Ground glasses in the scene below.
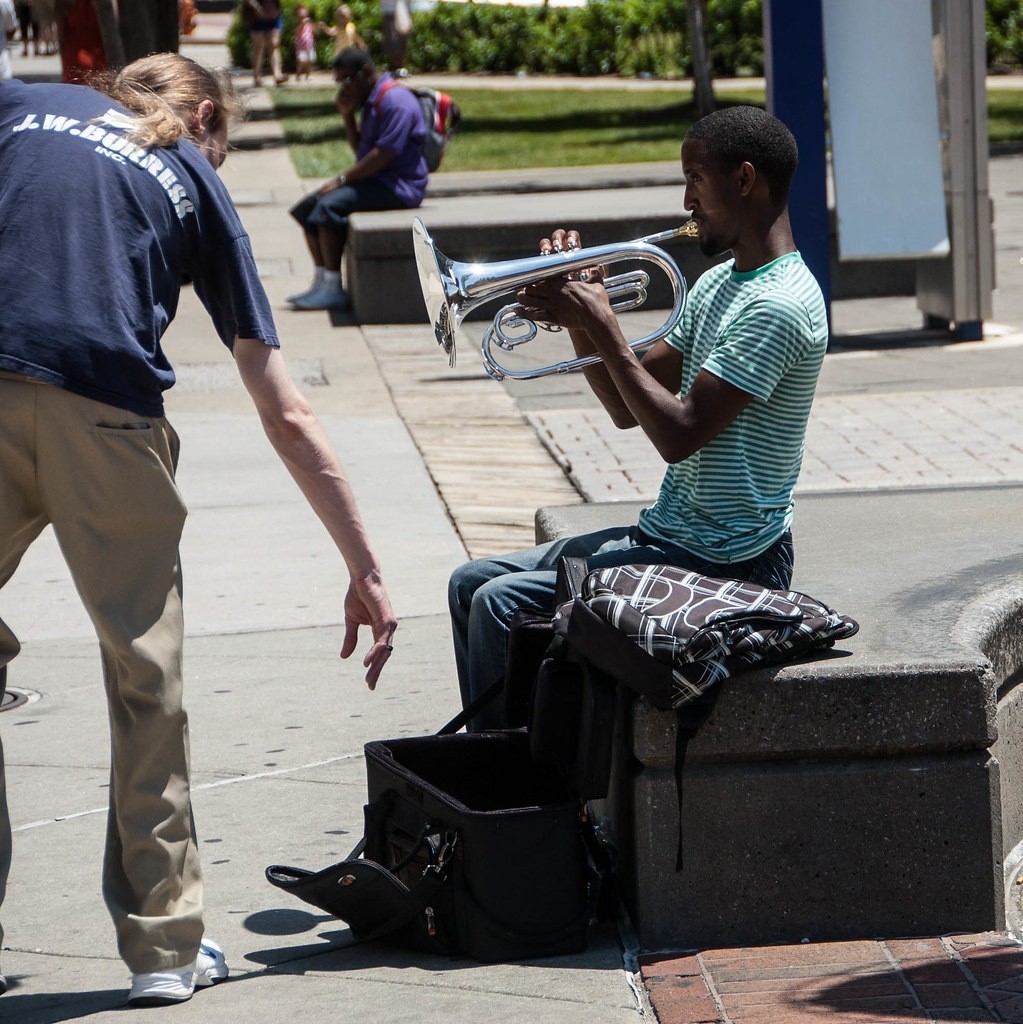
[337,64,364,87]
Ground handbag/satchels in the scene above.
[569,558,859,715]
[266,598,620,961]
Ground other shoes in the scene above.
[288,281,347,310]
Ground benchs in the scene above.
[342,158,732,323]
[537,481,1023,950]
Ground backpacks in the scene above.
[373,80,458,172]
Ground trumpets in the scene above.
[406,216,701,382]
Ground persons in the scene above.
[1,53,397,1000]
[1,0,59,57]
[289,49,431,310]
[447,106,827,731]
[247,1,412,87]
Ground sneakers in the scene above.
[127,938,230,1002]
